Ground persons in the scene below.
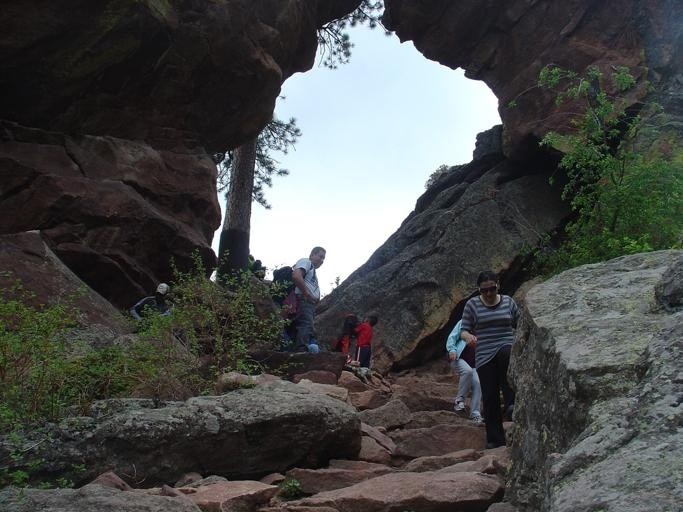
[284,247,326,351]
[460,271,520,449]
[446,319,483,423]
[129,283,176,323]
[352,315,378,367]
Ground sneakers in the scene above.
[470,410,486,424]
[454,395,465,412]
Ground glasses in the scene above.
[480,287,497,292]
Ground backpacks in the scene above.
[270,258,314,304]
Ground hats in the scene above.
[156,283,170,295]
